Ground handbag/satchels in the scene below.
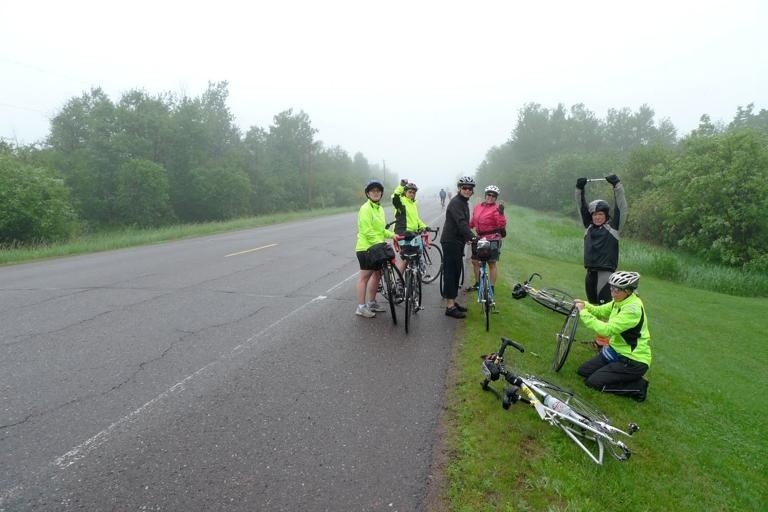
[365,244,395,268]
[472,240,500,262]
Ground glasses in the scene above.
[610,285,622,292]
[487,193,496,197]
[462,186,472,190]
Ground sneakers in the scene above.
[445,302,468,319]
[356,305,376,318]
[366,302,386,312]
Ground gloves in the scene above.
[605,174,620,186]
[496,204,504,214]
[577,177,588,187]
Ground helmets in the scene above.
[404,182,418,190]
[608,270,640,290]
[365,181,384,193]
[589,199,609,215]
[484,185,501,195]
[457,176,475,188]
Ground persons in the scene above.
[440,177,474,319]
[439,188,446,206]
[463,186,506,293]
[391,179,430,283]
[574,272,652,402]
[448,190,452,199]
[351,181,405,319]
[575,173,627,305]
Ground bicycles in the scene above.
[378,221,465,333]
[512,272,581,317]
[478,335,639,467]
[465,233,502,332]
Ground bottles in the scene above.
[543,393,570,419]
